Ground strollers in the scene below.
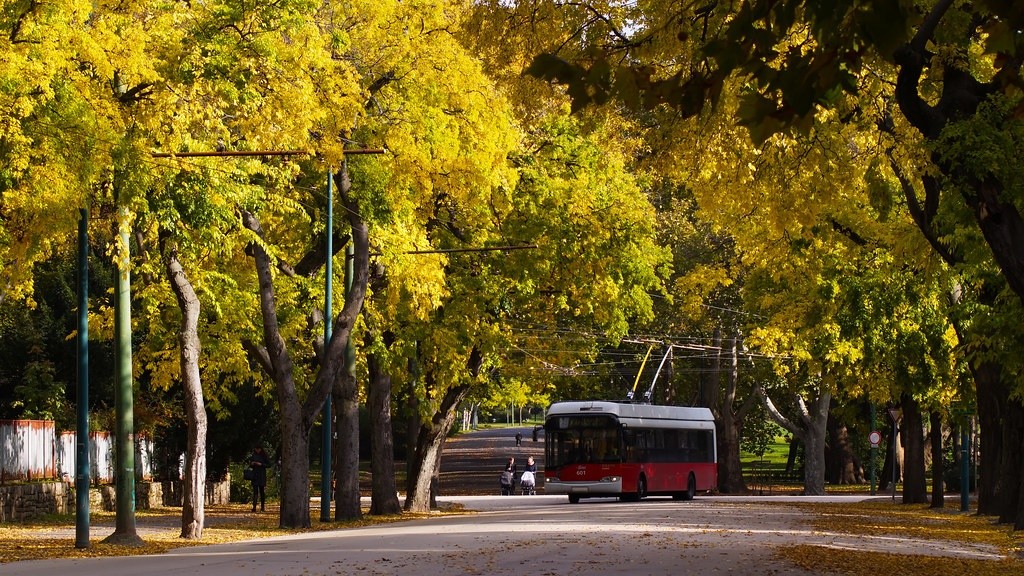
[520,471,536,495]
[500,471,512,495]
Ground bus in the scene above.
[543,402,718,503]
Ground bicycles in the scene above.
[516,439,521,450]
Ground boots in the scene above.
[261,502,264,511]
[251,503,256,511]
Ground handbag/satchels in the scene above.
[243,466,258,480]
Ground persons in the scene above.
[249,459,267,512]
[523,456,537,495]
[505,457,517,495]
[515,432,522,447]
